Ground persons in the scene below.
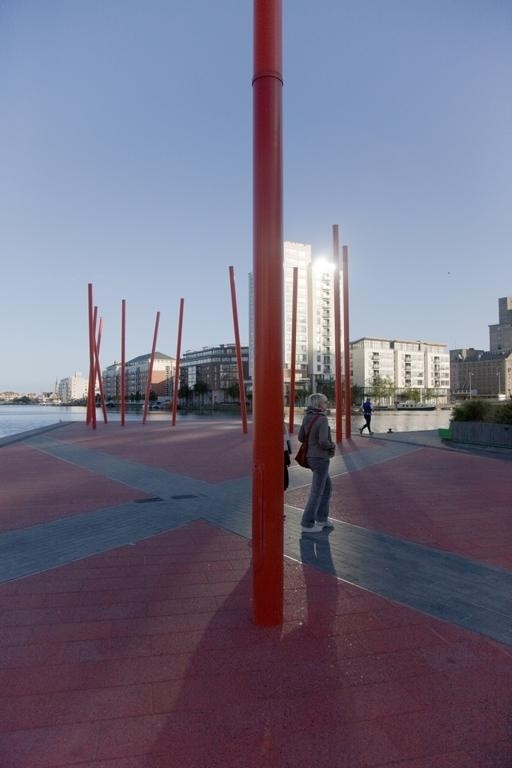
[283,410,292,517]
[298,393,336,532]
[358,397,374,435]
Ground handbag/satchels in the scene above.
[294,441,311,468]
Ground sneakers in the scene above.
[369,432,374,435]
[359,429,362,436]
[302,526,323,532]
[315,521,332,527]
